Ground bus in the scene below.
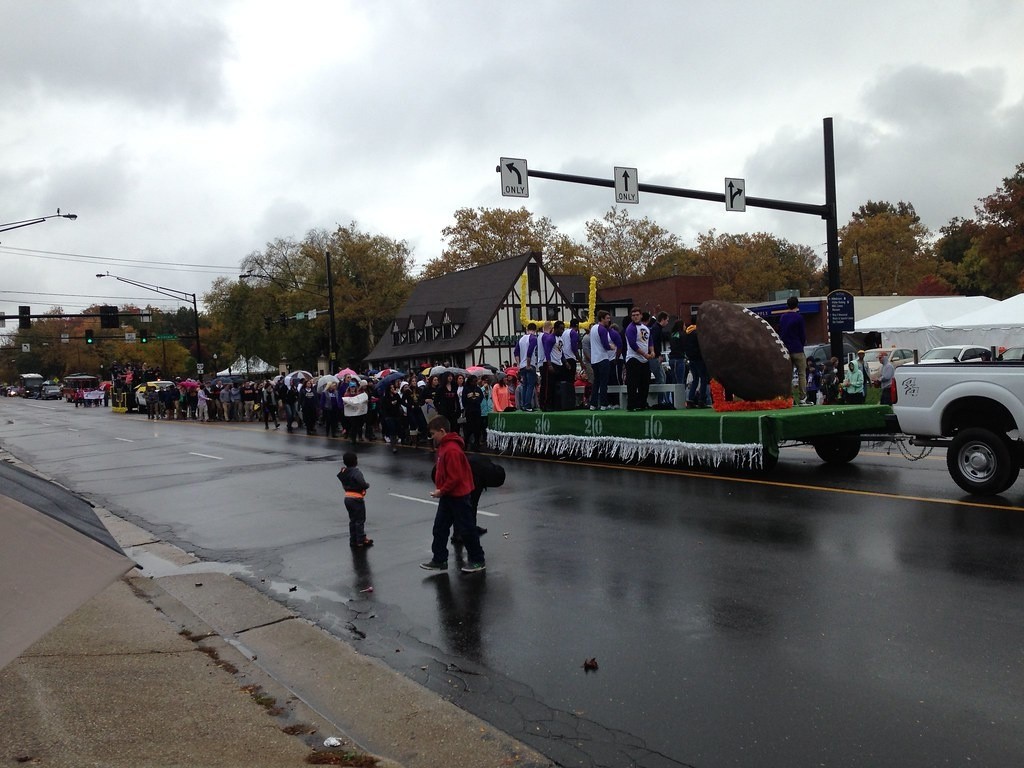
[63,373,100,404]
[19,374,44,399]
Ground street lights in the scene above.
[96,270,205,384]
[238,270,339,376]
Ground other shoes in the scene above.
[698,402,712,408]
[350,538,374,548]
[477,526,487,535]
[451,534,463,543]
[590,405,596,411]
[686,400,697,408]
[265,420,432,452]
[600,405,607,410]
[522,406,538,411]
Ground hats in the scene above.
[876,352,887,358]
[857,350,865,355]
[359,379,369,386]
[497,372,506,382]
[417,381,426,388]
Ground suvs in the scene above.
[802,343,858,371]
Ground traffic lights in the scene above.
[85,329,94,345]
[139,329,148,343]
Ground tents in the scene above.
[215,353,279,380]
[854,293,1024,360]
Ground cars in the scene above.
[134,380,175,414]
[903,345,992,365]
[1000,346,1024,362]
[41,386,63,400]
[844,347,923,384]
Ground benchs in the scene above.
[576,384,686,410]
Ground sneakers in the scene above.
[420,557,449,570]
[461,559,485,572]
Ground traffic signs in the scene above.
[499,157,529,197]
[613,166,640,204]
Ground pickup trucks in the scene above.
[891,359,1024,498]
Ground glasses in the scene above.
[346,376,351,379]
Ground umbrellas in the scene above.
[338,368,357,377]
[285,370,312,379]
[373,372,409,391]
[442,367,467,375]
[317,375,339,393]
[374,369,399,378]
[465,366,495,376]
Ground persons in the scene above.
[74,382,111,408]
[513,323,538,412]
[581,307,677,411]
[336,452,373,547]
[420,415,488,572]
[670,316,713,409]
[537,318,587,412]
[112,358,541,453]
[778,296,815,407]
[804,349,894,407]
